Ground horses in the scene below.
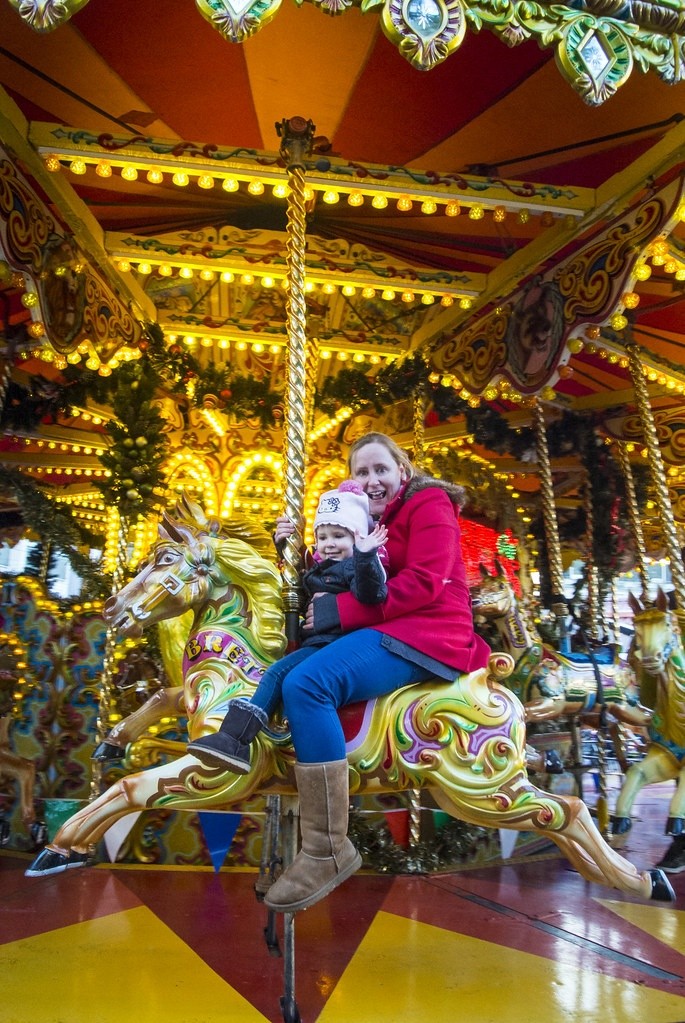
[468,559,656,775]
[613,584,685,835]
[25,491,677,905]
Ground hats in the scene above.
[313,479,370,539]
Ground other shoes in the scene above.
[654,833,685,873]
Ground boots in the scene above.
[263,759,362,913]
[187,698,268,774]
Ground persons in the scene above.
[185,478,393,776]
[254,432,492,913]
